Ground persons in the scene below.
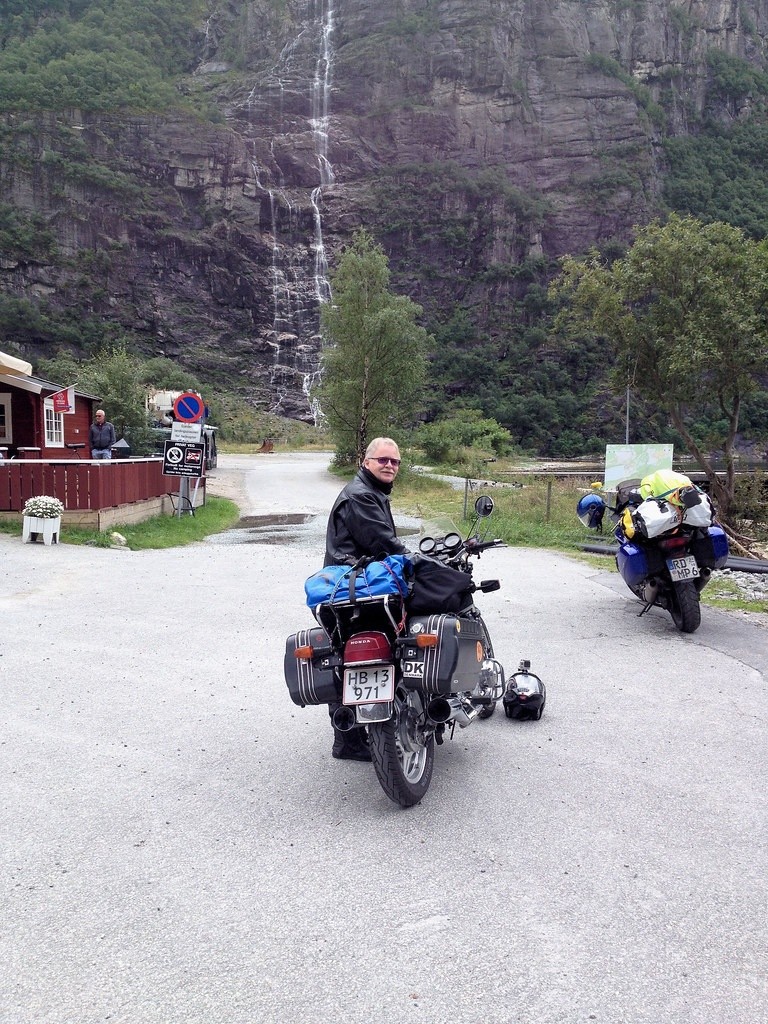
[323,438,411,763]
[88,410,116,459]
[587,504,600,527]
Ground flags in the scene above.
[53,385,75,414]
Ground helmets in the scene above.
[503,659,546,721]
[577,494,606,533]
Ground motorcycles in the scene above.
[576,478,729,633]
[284,495,508,807]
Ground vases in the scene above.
[22,516,61,546]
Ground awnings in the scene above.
[0,351,32,376]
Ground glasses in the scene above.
[95,414,101,417]
[368,457,401,467]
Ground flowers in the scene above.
[21,494,65,519]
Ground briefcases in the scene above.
[402,614,485,693]
[284,627,343,708]
